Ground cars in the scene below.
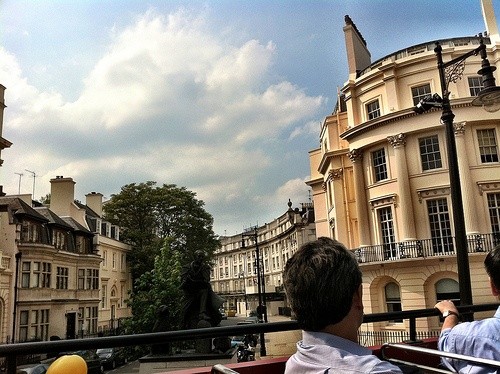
[15,364,51,374]
[96,347,125,369]
[230,335,246,346]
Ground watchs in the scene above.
[441,310,460,319]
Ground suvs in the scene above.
[56,350,104,374]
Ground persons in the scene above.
[434,243,500,374]
[283,235,404,374]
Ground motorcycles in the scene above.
[237,343,257,362]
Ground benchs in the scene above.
[381,343,500,374]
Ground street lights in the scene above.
[433,34,500,325]
[242,234,267,321]
